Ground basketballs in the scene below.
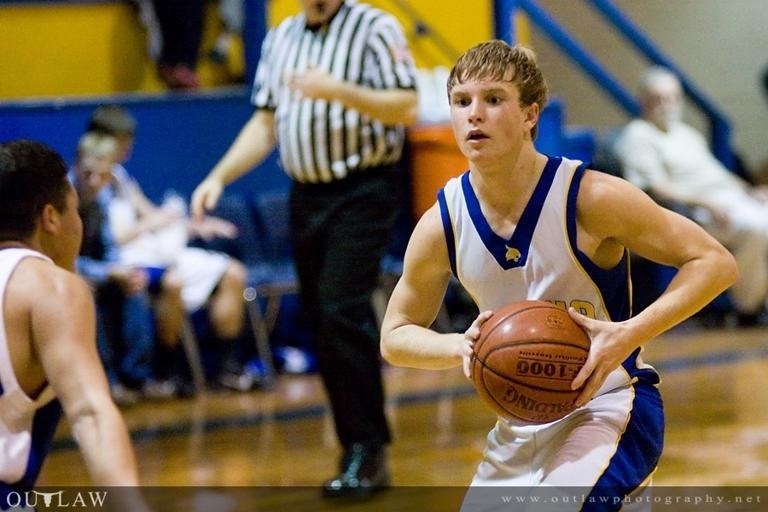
[468,300,591,425]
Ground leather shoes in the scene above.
[324,440,391,499]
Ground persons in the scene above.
[191,0,420,505]
[66,108,262,406]
[132,0,211,92]
[610,66,768,329]
[0,138,149,512]
[377,40,740,512]
